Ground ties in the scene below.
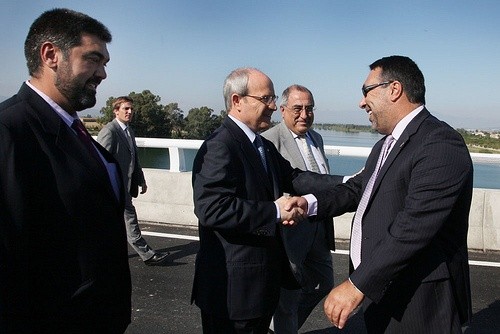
[349,135,393,270]
[125,127,135,170]
[299,134,321,174]
[255,135,268,176]
[71,118,107,172]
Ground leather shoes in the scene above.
[144,252,169,266]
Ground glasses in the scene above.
[283,104,316,114]
[240,95,278,106]
[362,80,401,98]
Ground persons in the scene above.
[190,68,366,334]
[97,97,170,265]
[258,84,358,334]
[283,56,474,334]
[0,9,132,334]
